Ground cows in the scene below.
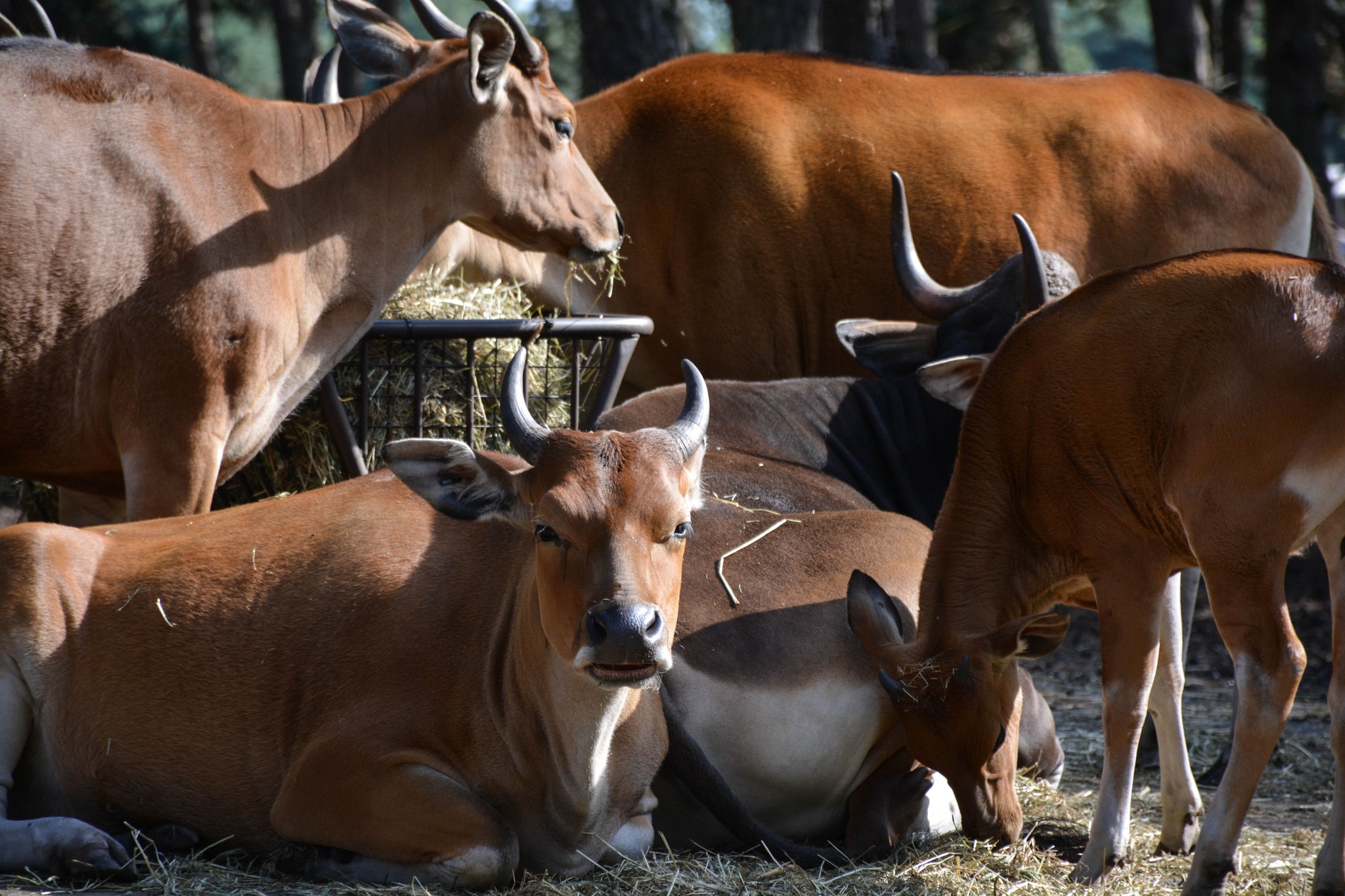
[0,0,621,529]
[271,0,1344,896]
[0,348,710,889]
